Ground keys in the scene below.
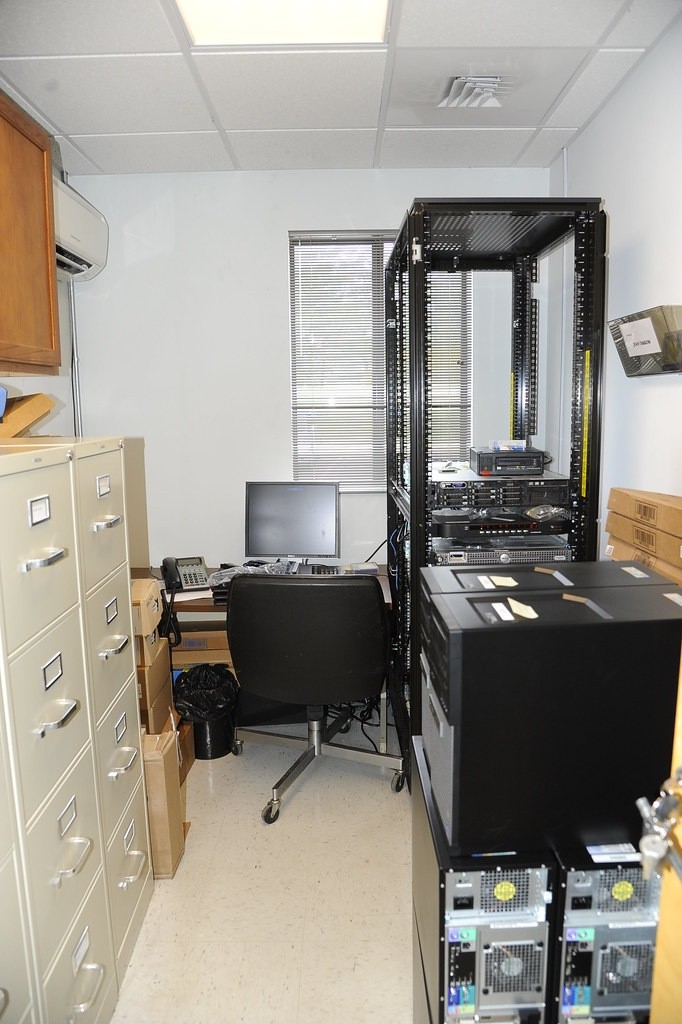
[639,834,668,880]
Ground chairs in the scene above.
[224,573,413,824]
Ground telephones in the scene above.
[160,556,211,592]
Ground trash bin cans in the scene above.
[173,663,237,762]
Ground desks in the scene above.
[129,564,229,611]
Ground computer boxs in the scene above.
[412,559,682,1024]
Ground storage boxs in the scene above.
[130,575,230,876]
[0,392,54,439]
[605,488,682,589]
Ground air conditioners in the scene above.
[53,172,109,284]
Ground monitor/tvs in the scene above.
[245,482,340,564]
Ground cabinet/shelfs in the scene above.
[0,436,151,1024]
[0,86,61,378]
